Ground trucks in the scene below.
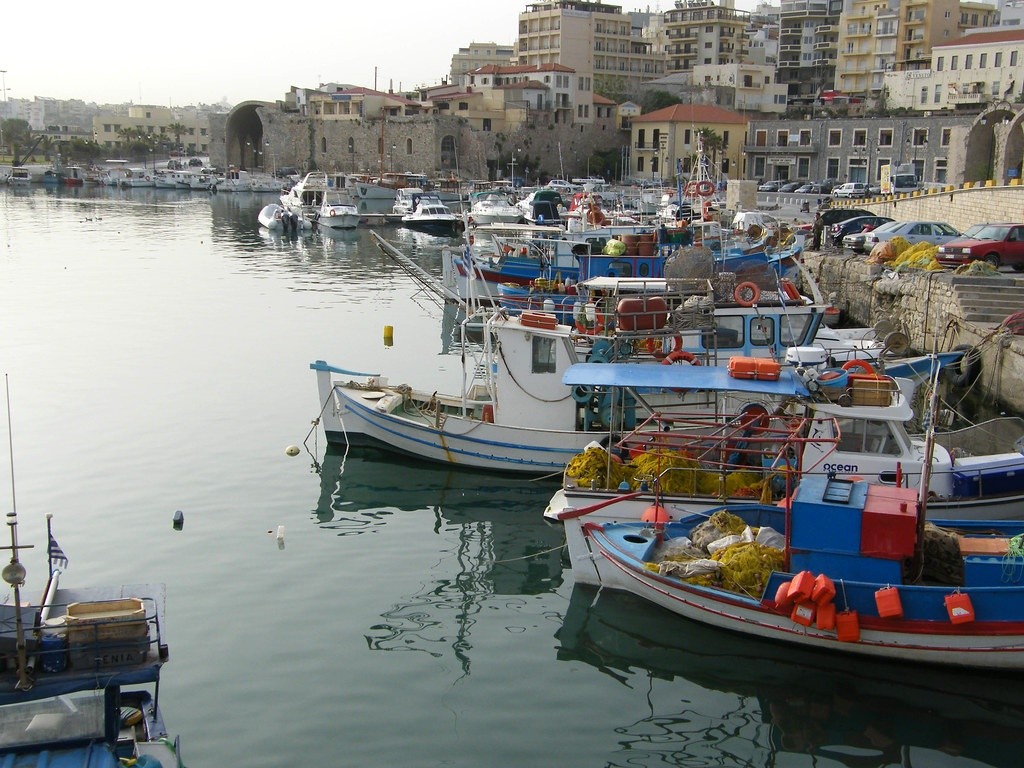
[880,163,920,200]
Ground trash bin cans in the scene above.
[336,176,346,188]
[802,202,809,211]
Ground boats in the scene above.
[0,371,186,768]
[6,166,33,187]
[44,125,1024,673]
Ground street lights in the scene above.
[348,142,355,174]
[387,141,397,173]
[905,127,931,181]
[861,137,881,198]
[511,145,522,188]
[737,140,747,180]
[258,133,271,173]
[852,143,867,183]
[246,138,256,175]
[655,141,664,187]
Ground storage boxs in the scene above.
[850,378,893,405]
[954,451,1024,498]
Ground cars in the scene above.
[820,207,877,226]
[840,220,942,255]
[935,222,1024,274]
[863,220,964,253]
[829,215,897,246]
